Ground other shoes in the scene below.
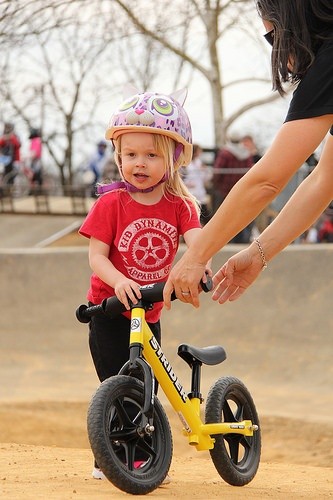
[92,460,171,485]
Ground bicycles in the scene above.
[75,269,262,496]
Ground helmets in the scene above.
[104,92,193,167]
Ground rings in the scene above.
[182,292,190,295]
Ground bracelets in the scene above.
[255,239,267,270]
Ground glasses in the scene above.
[263,27,275,47]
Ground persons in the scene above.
[78,93,212,481]
[0,124,45,195]
[162,0,333,309]
[89,141,111,198]
[184,136,333,241]
